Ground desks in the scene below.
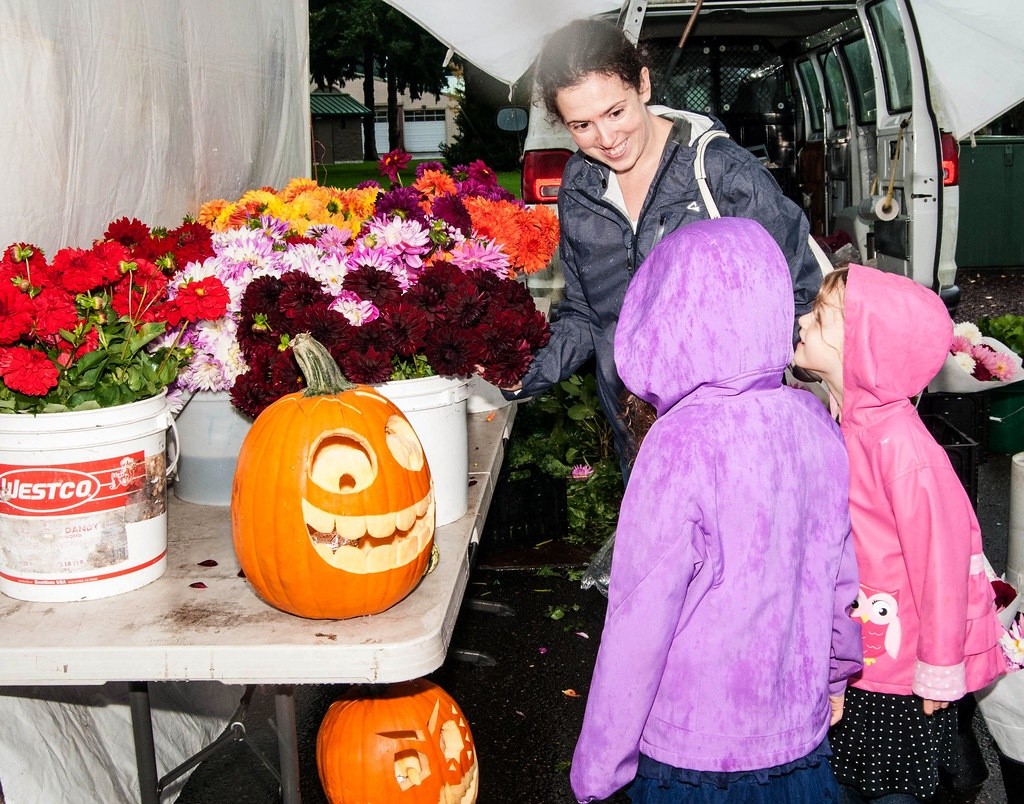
[0,404,516,804]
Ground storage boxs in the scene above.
[921,413,977,511]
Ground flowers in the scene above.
[0,149,560,412]
[931,322,1024,391]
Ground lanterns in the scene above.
[316,678,479,804]
[233,332,434,617]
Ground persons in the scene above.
[569,218,861,803]
[470,19,825,493]
[795,262,1005,804]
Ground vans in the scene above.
[495,0,960,319]
